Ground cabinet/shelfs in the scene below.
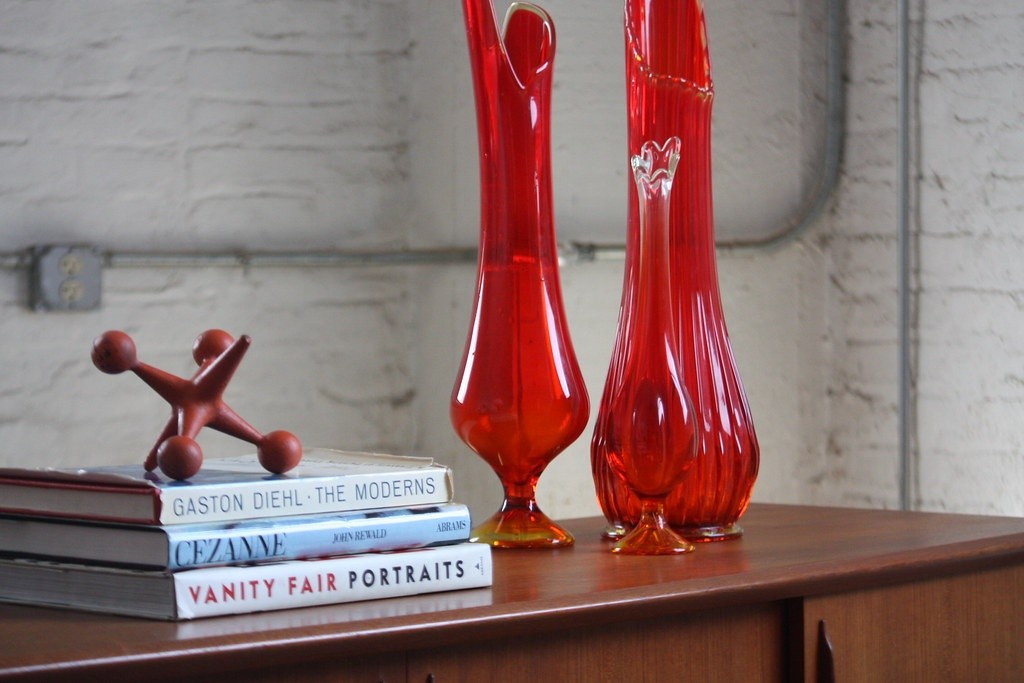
[0,504,1024,683]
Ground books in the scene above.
[0,447,493,622]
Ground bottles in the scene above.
[609,135,693,556]
[589,1,761,544]
[448,1,590,551]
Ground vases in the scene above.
[591,0,756,555]
[450,2,592,551]
